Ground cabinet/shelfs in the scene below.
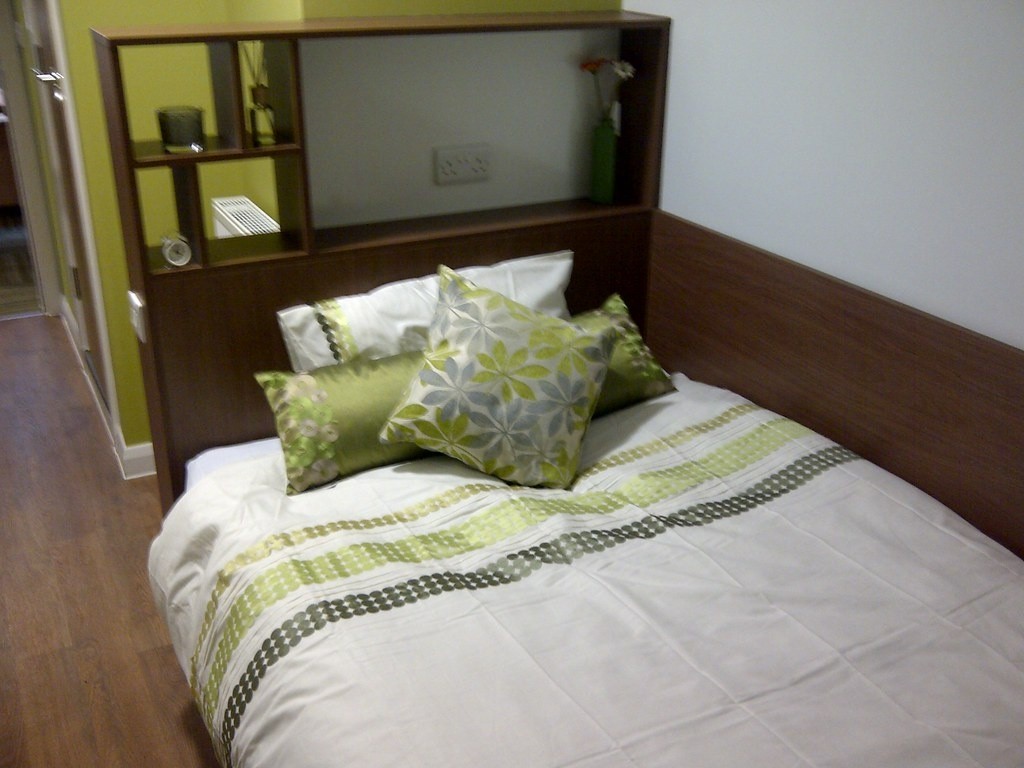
[91,9,672,517]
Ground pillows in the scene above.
[254,294,677,496]
[277,249,574,373]
[379,264,626,489]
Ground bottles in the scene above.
[247,84,283,150]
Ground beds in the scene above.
[146,372,1023,767]
